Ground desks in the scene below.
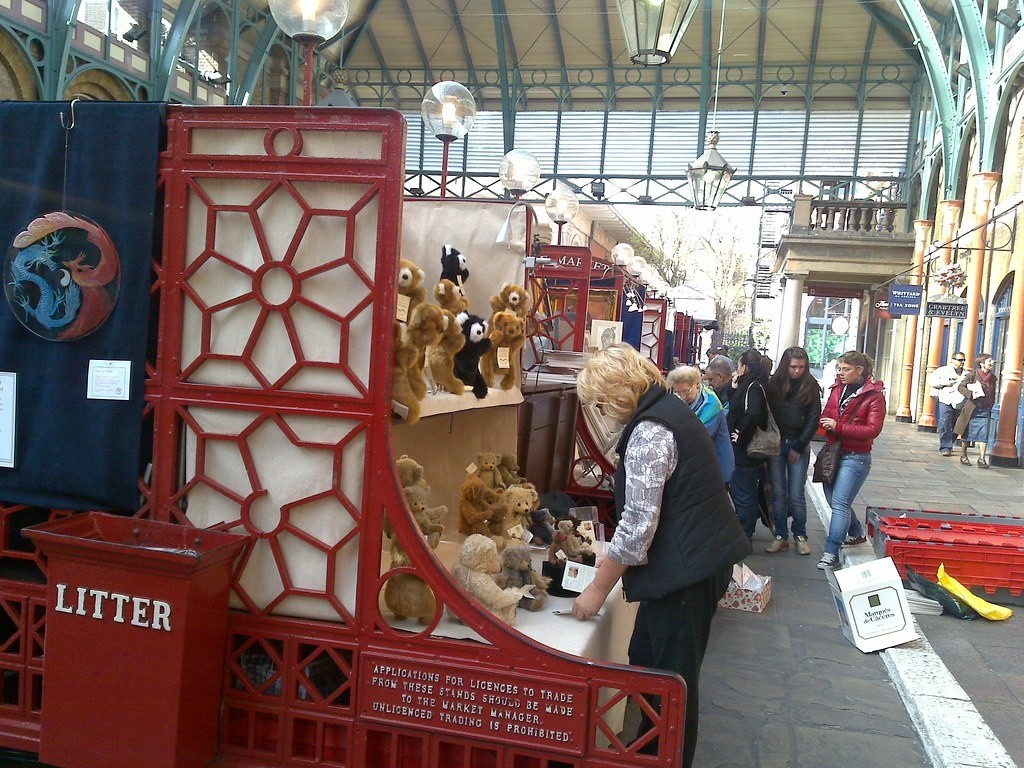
[378,540,641,749]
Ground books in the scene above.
[905,590,944,616]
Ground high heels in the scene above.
[977,458,990,468]
[960,456,971,466]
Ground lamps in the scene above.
[639,196,651,203]
[204,71,232,84]
[623,279,648,312]
[545,187,579,245]
[614,0,701,68]
[685,0,738,212]
[409,188,424,196]
[420,81,478,197]
[590,181,605,196]
[613,243,676,306]
[498,149,540,201]
[267,0,349,105]
[741,197,756,205]
[122,23,147,43]
[495,200,540,258]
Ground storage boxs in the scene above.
[864,505,1024,607]
[823,556,917,654]
[717,574,771,614]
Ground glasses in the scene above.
[953,357,966,362]
[837,365,861,373]
[596,401,604,409]
[676,384,695,396]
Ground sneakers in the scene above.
[795,536,811,555]
[766,534,789,552]
[841,534,868,548]
[817,552,839,570]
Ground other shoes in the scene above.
[941,451,950,455]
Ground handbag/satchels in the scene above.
[906,564,980,621]
[935,562,1013,621]
[812,442,841,485]
[744,381,782,459]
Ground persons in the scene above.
[816,351,886,570]
[701,345,777,537]
[572,342,752,768]
[764,346,823,555]
[665,365,735,484]
[932,352,970,456]
[953,354,998,467]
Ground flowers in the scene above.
[933,264,967,289]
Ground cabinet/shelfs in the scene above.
[0,105,698,768]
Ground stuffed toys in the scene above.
[385,451,596,625]
[391,244,531,425]
